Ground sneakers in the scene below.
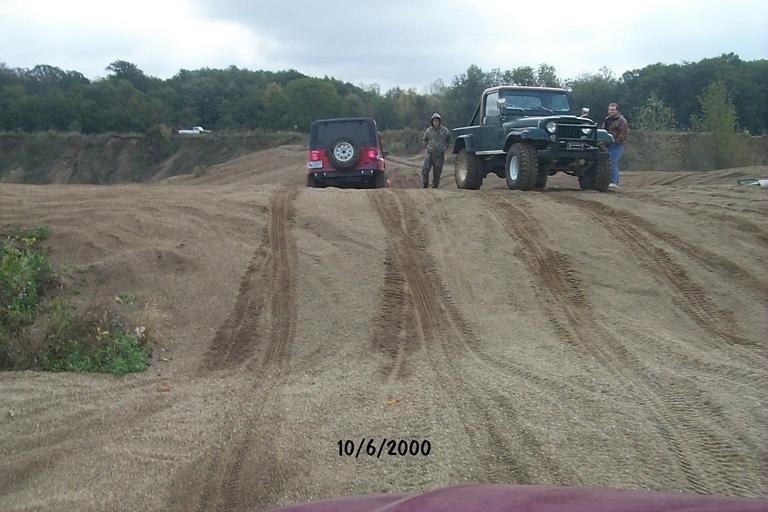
[608,182,618,188]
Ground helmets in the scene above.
[431,113,441,126]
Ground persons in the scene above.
[603,103,629,188]
[421,113,451,188]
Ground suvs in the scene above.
[305,116,388,189]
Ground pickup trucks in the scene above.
[179,126,211,136]
[449,85,616,196]
